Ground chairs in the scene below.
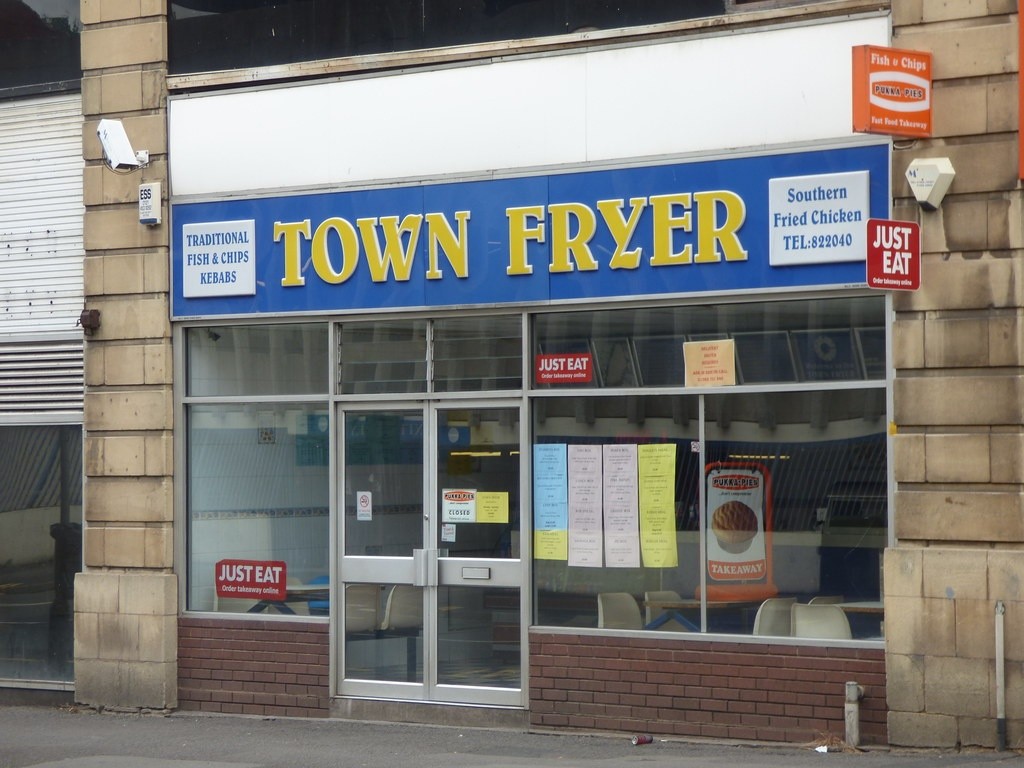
[751,593,855,642]
[595,590,692,634]
[307,574,421,681]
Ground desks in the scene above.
[825,602,886,640]
[249,584,327,615]
[645,599,763,635]
